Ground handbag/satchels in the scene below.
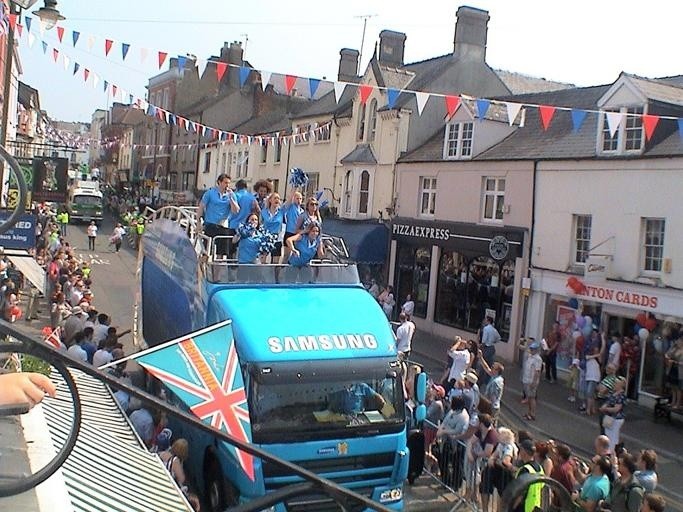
[602,414,615,430]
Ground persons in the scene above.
[196,173,324,283]
[365,275,415,364]
[379,329,682,512]
[329,383,385,414]
[544,321,561,383]
[0,162,201,512]
[477,316,502,361]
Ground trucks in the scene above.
[64,167,105,227]
[131,201,411,512]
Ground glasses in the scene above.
[310,202,318,206]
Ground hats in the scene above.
[157,429,172,446]
[518,440,536,452]
[62,289,98,319]
[431,384,446,398]
[463,372,478,384]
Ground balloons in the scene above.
[620,314,655,373]
[566,278,593,350]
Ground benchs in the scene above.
[654,377,683,421]
